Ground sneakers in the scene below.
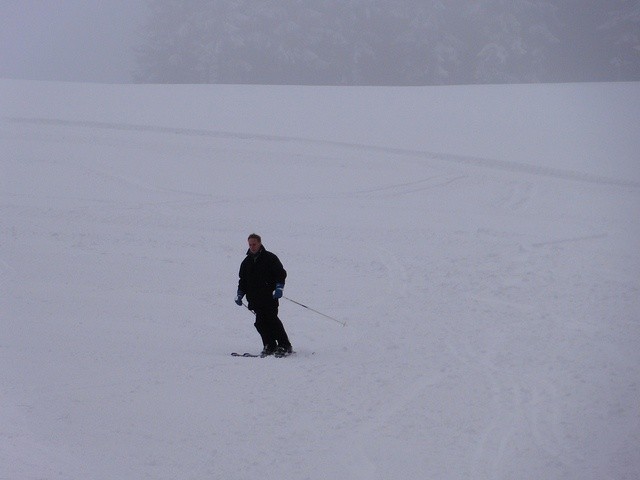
[261,343,277,355]
[275,345,291,356]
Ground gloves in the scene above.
[272,284,284,298]
[236,291,245,306]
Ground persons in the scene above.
[235,233,293,358]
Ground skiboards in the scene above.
[231,351,295,358]
